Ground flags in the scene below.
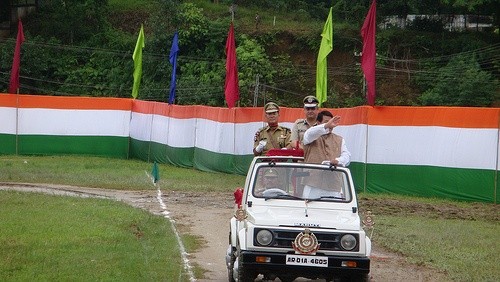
[132,24,145,99]
[225,24,240,109]
[361,0,376,106]
[9,18,25,94]
[316,7,333,108]
[169,31,179,104]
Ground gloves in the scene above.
[256,140,266,153]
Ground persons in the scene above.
[291,95,319,197]
[253,102,296,193]
[303,111,351,199]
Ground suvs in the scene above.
[226,149,373,282]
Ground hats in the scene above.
[264,102,280,113]
[303,95,319,107]
[263,168,280,177]
